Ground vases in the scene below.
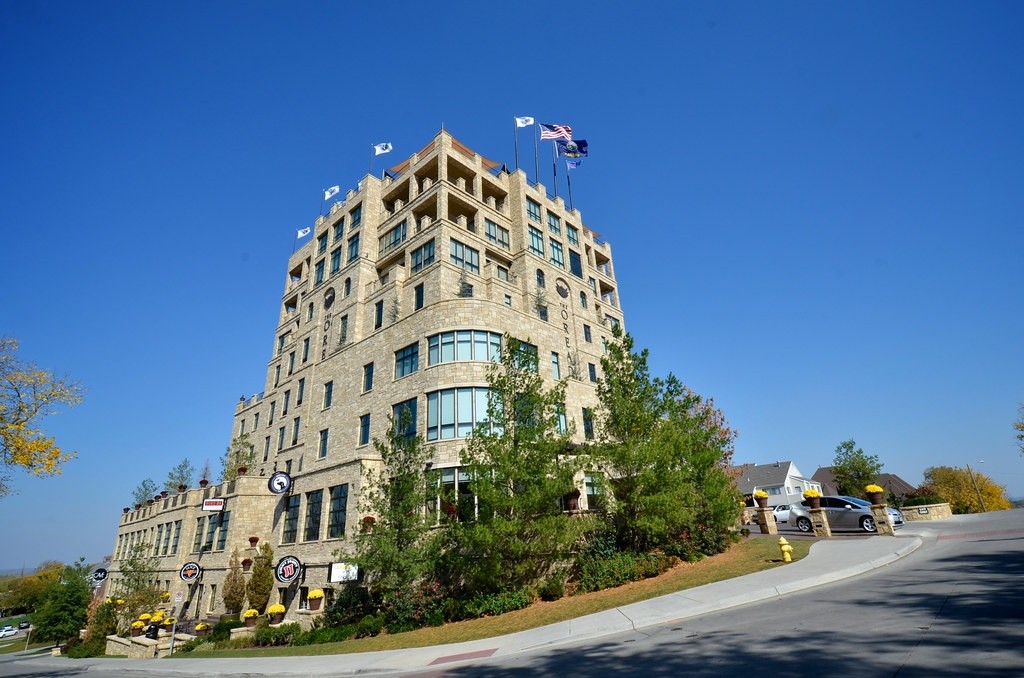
[250,542,257,547]
[239,472,245,476]
[132,629,141,637]
[197,630,206,637]
[180,488,185,492]
[363,524,371,533]
[309,598,321,610]
[162,598,168,603]
[139,619,149,626]
[148,502,152,506]
[806,498,820,509]
[162,494,166,498]
[865,493,881,505]
[270,613,282,624]
[566,498,578,510]
[166,625,173,632]
[135,506,139,510]
[151,622,161,625]
[201,484,206,488]
[155,498,159,502]
[755,498,767,508]
[245,618,255,627]
[124,510,128,513]
[243,565,250,571]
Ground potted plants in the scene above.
[220,546,246,622]
[240,394,245,401]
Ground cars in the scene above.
[18,620,30,629]
[751,504,791,524]
[0,628,19,638]
[4,624,14,631]
[789,496,905,533]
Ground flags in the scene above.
[325,185,339,201]
[374,142,392,156]
[297,226,311,239]
[538,123,572,142]
[567,160,584,169]
[515,116,534,128]
[554,139,589,159]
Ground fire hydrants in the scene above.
[777,535,794,563]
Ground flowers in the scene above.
[106,599,113,604]
[116,599,125,604]
[164,617,177,625]
[802,490,821,498]
[147,499,154,503]
[243,609,259,618]
[864,484,884,493]
[248,537,259,542]
[199,479,208,484]
[362,516,375,524]
[753,490,768,499]
[178,485,187,489]
[160,592,170,598]
[307,589,324,599]
[123,507,130,511]
[155,495,161,499]
[131,621,145,629]
[135,504,142,507]
[195,623,207,630]
[237,467,247,472]
[138,610,165,622]
[241,558,253,566]
[160,491,168,496]
[267,603,286,614]
[564,488,581,498]
